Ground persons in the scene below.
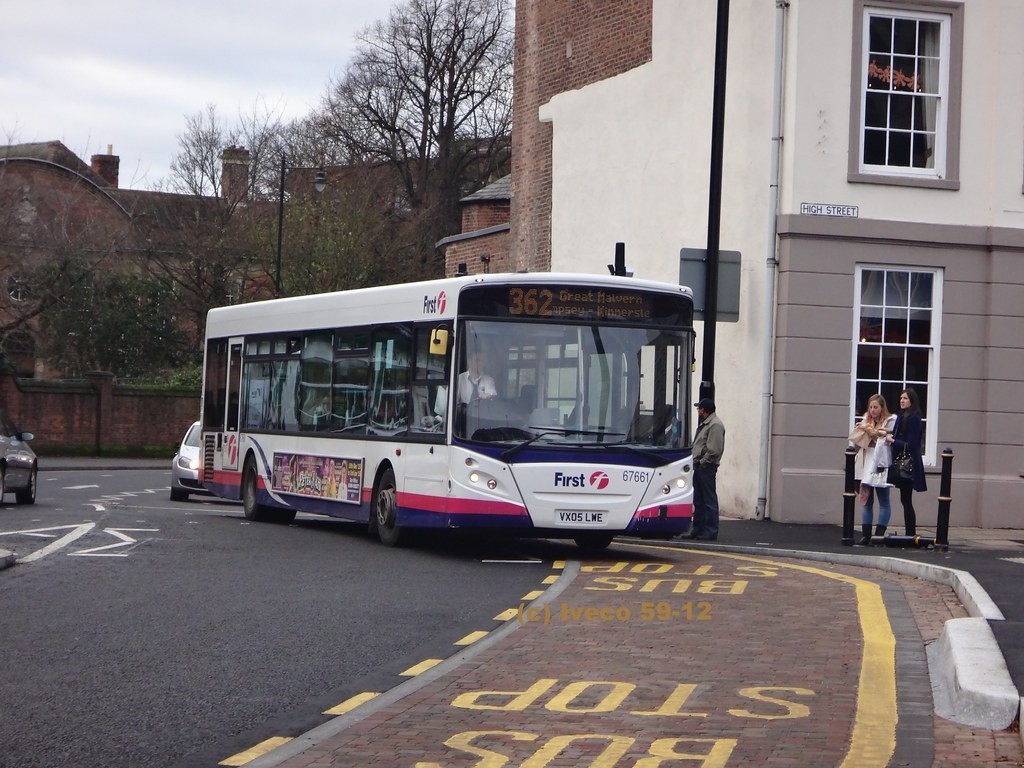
[888,388,927,536]
[326,457,338,500]
[662,401,683,446]
[434,345,498,420]
[847,393,900,542]
[691,400,726,541]
[337,461,349,501]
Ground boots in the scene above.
[871,524,887,547]
[858,524,873,545]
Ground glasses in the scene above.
[697,407,701,410]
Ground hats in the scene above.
[693,398,715,413]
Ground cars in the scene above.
[0,409,39,506]
[169,420,217,503]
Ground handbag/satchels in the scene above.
[892,442,916,483]
[877,437,891,468]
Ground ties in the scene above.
[468,376,481,403]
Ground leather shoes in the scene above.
[681,532,717,540]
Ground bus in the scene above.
[198,270,695,558]
